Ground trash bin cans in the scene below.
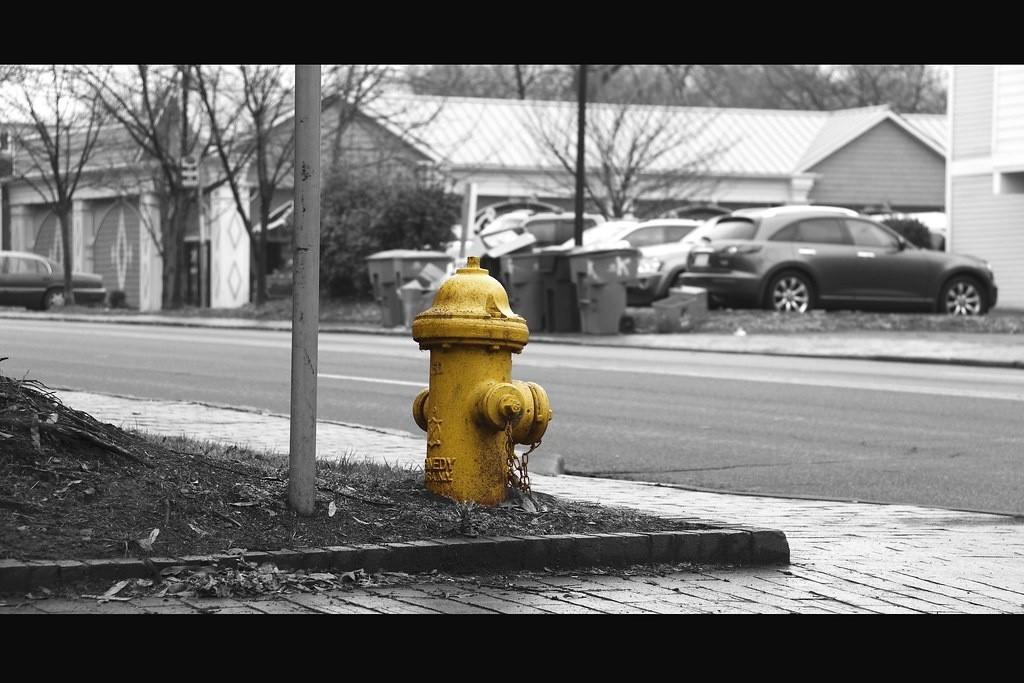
[391,250,455,329]
[567,247,641,335]
[498,252,546,333]
[366,248,405,327]
[542,251,584,332]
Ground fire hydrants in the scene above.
[407,253,555,505]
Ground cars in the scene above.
[1,248,107,310]
[451,198,707,294]
[676,201,1000,319]
